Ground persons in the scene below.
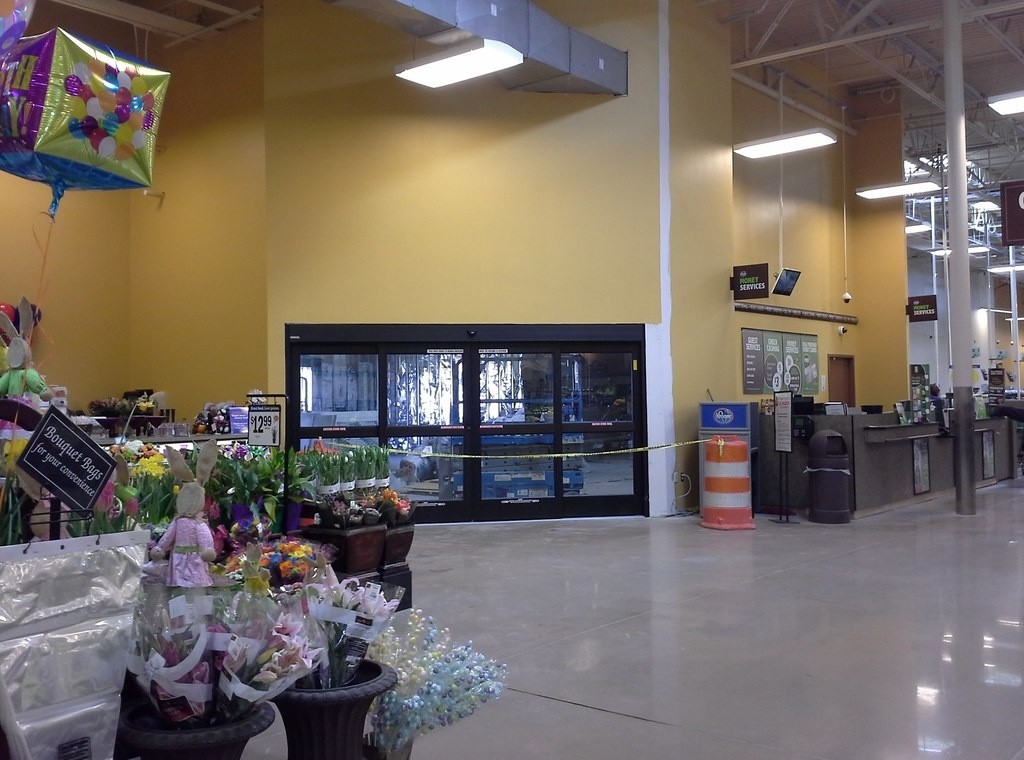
[929,383,941,400]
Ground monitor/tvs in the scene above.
[861,405,883,414]
[771,268,802,296]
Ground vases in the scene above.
[302,523,386,576]
[375,525,414,573]
[268,658,399,760]
[77,424,93,436]
[117,696,276,760]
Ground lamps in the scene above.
[394,36,523,89]
[855,100,941,201]
[904,195,1024,321]
[988,90,1024,115]
[732,51,837,159]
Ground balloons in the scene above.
[0,26,171,214]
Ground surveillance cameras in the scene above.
[842,292,851,303]
[839,326,848,335]
[1010,341,1013,345]
[997,340,1000,344]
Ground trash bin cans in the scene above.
[808,429,850,523]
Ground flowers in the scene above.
[131,538,399,728]
[0,390,391,485]
[63,472,139,536]
[322,487,417,525]
[228,516,273,550]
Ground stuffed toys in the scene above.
[149,439,220,588]
[15,457,70,541]
[0,296,54,402]
